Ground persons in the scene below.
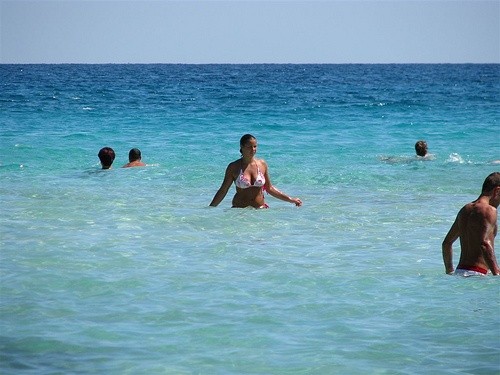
[441,172,500,278]
[120,148,145,168]
[98,147,115,169]
[415,141,428,157]
[208,134,303,209]
